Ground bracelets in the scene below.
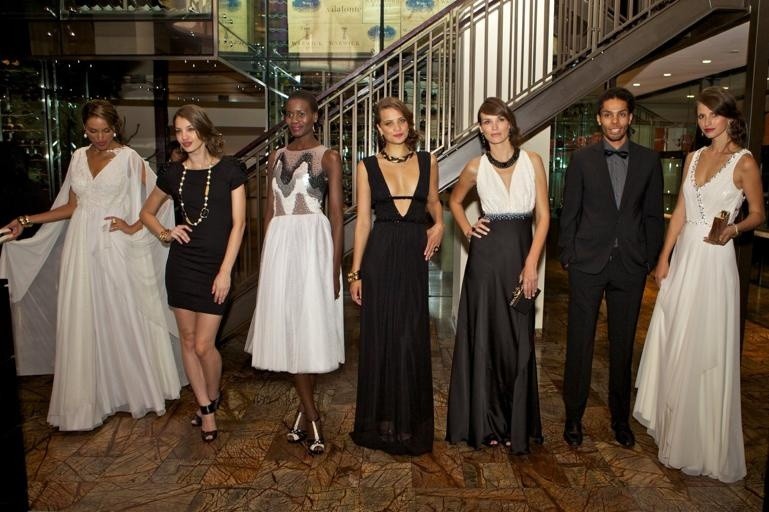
[348,275,360,283]
[347,270,361,277]
[159,230,171,242]
[18,215,26,228]
[732,223,738,238]
[25,215,32,227]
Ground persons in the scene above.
[139,104,248,442]
[632,86,766,485]
[445,96,550,455]
[557,86,664,447]
[157,141,183,177]
[0,100,190,431]
[243,89,345,455]
[347,96,445,457]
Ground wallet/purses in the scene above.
[707,210,731,241]
[508,284,542,316]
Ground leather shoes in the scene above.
[481,432,500,448]
[610,416,635,447]
[562,416,584,448]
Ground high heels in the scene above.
[198,400,217,442]
[305,413,327,455]
[286,407,308,443]
[190,390,222,427]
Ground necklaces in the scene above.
[487,147,520,168]
[381,149,415,163]
[179,162,212,227]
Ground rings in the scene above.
[434,247,440,253]
[113,218,116,224]
[472,226,475,231]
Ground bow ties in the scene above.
[603,149,630,160]
